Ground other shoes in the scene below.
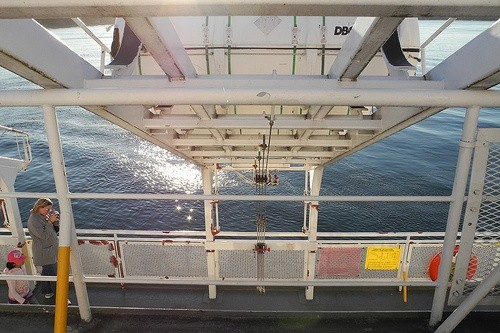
[45,292,55,298]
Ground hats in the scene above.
[7,249,27,264]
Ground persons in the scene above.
[2,250,39,305]
[27,198,72,305]
